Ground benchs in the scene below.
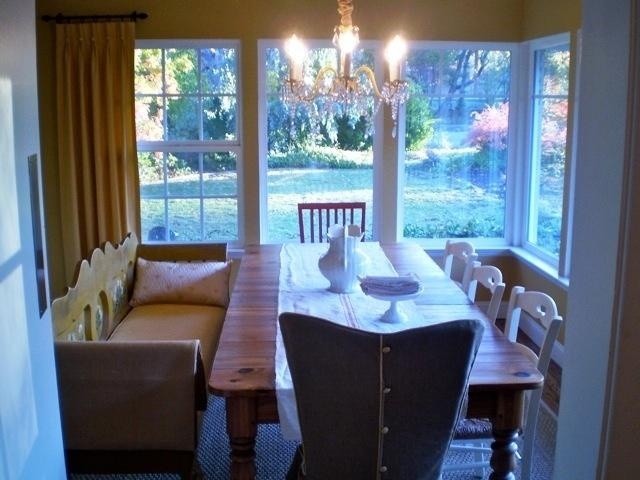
[50,233,226,450]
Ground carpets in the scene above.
[67,393,558,480]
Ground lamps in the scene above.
[277,0,410,139]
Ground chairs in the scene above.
[441,240,479,293]
[439,286,562,480]
[297,203,365,243]
[467,262,506,325]
[278,312,485,480]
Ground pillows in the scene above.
[129,257,233,309]
